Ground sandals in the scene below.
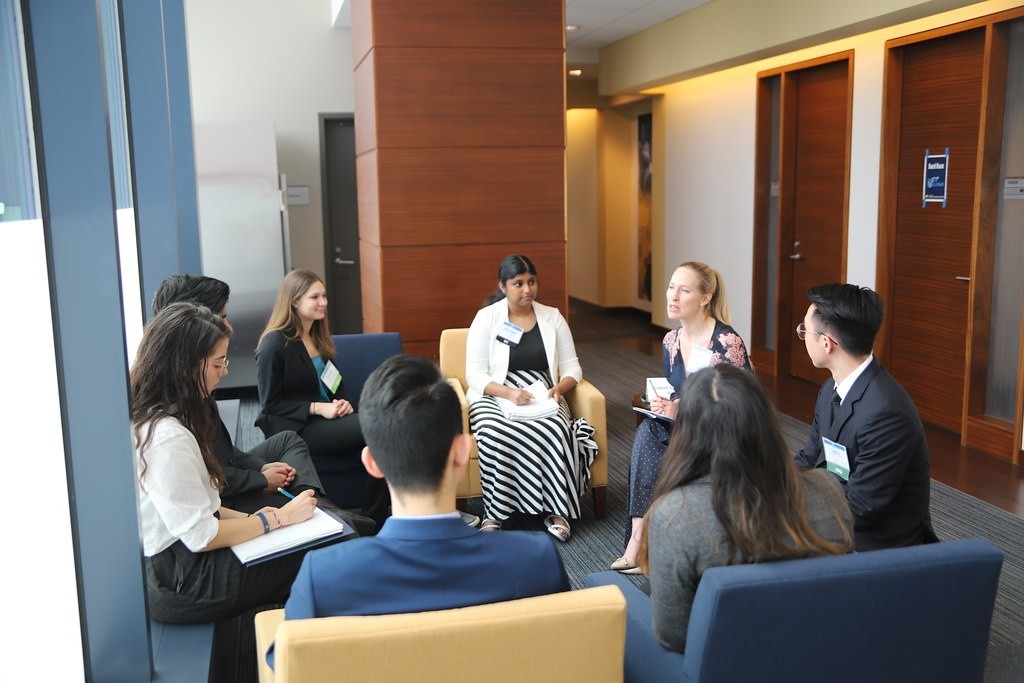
[543,514,571,542]
[479,514,501,532]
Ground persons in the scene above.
[254,269,385,504]
[634,364,854,656]
[791,285,939,553]
[266,354,572,672]
[129,274,377,625]
[466,254,599,543]
[609,262,751,576]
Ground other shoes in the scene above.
[611,557,646,574]
[309,496,377,534]
[454,506,481,527]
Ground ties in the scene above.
[828,386,842,428]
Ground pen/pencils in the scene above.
[517,382,535,399]
[277,487,294,499]
[649,380,658,397]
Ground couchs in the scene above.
[440,328,607,513]
[588,539,1004,683]
[254,585,625,682]
[326,333,402,410]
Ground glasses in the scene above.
[198,359,230,371]
[795,323,838,346]
